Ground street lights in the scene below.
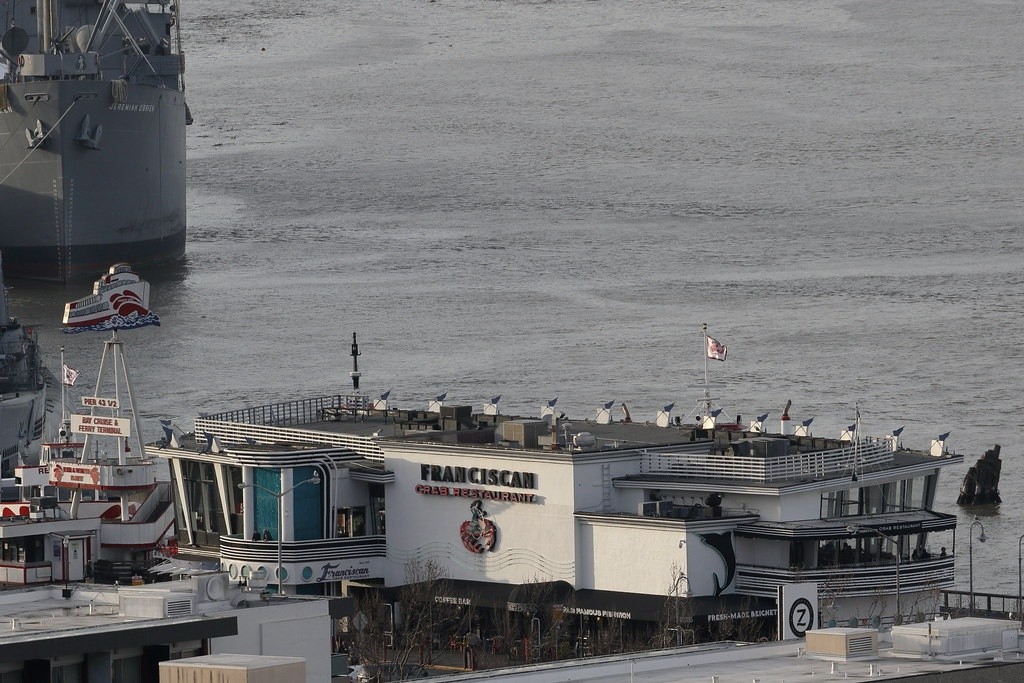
[676,577,695,646]
[970,522,990,616]
[237,477,321,595]
[847,526,900,626]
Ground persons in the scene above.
[818,542,948,566]
[252,529,273,542]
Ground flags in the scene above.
[63,364,80,386]
[706,335,727,361]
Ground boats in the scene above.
[0,264,48,479]
[1,0,193,288]
